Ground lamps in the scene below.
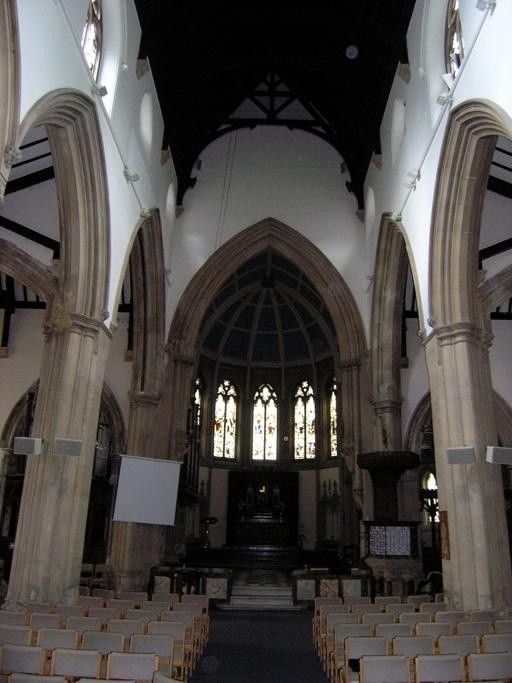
[390,0,497,221]
[58,0,150,219]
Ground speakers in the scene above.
[50,437,84,458]
[486,445,512,466]
[444,446,476,466]
[11,435,43,457]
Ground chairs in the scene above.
[0,587,210,683]
[80,564,112,589]
[312,593,512,683]
